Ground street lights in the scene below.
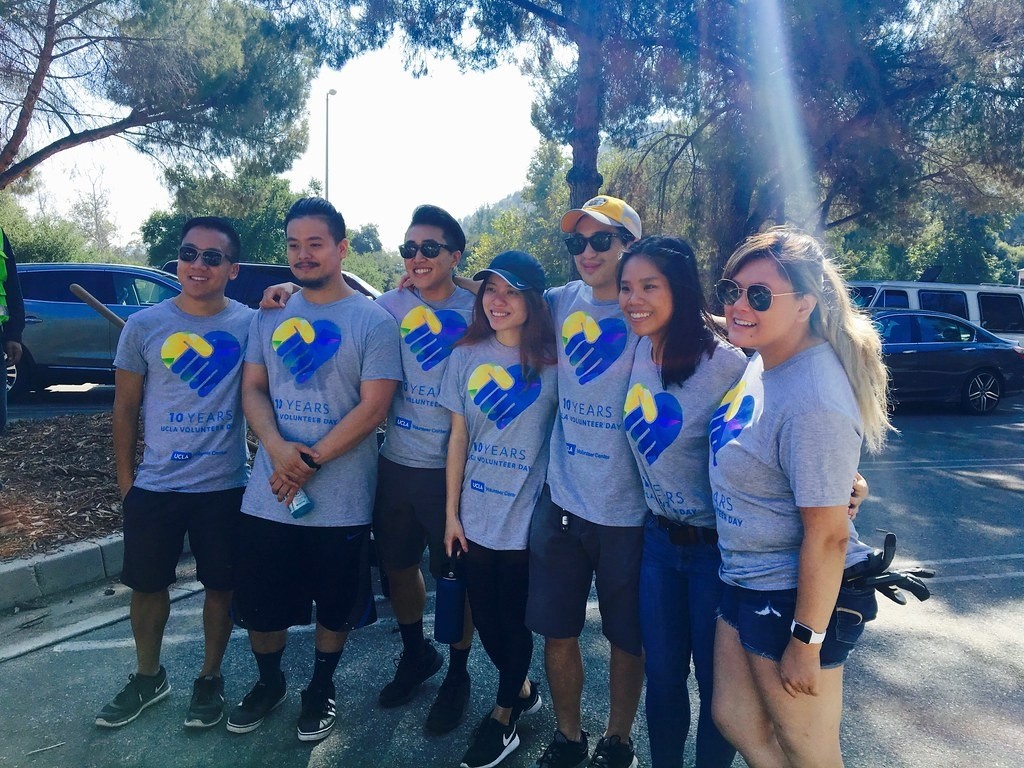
[325,88,337,202]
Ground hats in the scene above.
[560,195,641,240]
[473,251,546,295]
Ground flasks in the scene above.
[434,542,466,643]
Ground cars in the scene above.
[6,261,182,404]
[149,259,383,313]
[869,309,1024,417]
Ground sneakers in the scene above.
[535,729,590,768]
[587,733,639,768]
[185,671,225,726]
[426,671,470,733]
[461,713,520,768]
[378,638,442,707]
[97,665,171,726]
[226,671,287,733]
[480,682,541,726]
[296,681,336,740]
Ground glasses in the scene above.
[714,278,806,311]
[564,232,634,255]
[177,246,235,266]
[398,239,452,259]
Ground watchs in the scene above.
[791,619,826,644]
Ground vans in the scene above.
[848,279,1024,347]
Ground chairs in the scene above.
[938,327,959,342]
[889,325,905,343]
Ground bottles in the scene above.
[283,486,314,518]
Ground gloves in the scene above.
[858,532,935,606]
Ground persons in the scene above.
[394,192,725,768]
[615,236,867,768]
[259,203,478,737]
[224,198,405,742]
[702,229,889,767]
[94,217,259,730]
[435,250,557,768]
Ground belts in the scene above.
[651,515,719,546]
